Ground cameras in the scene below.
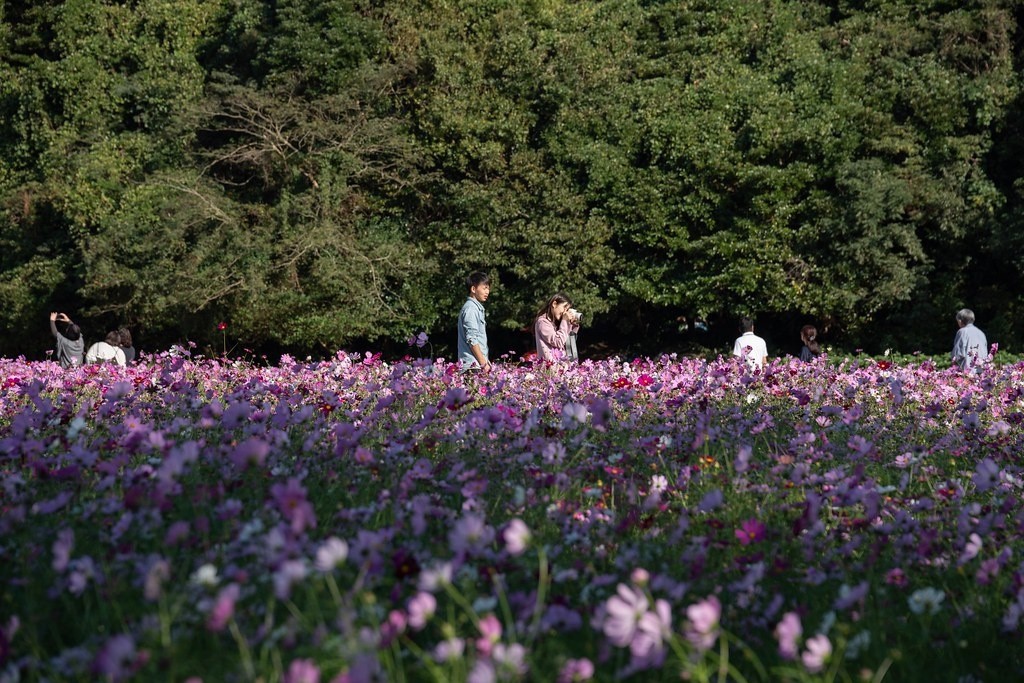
[568,308,582,322]
[56,314,64,320]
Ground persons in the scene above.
[535,291,577,365]
[457,270,492,376]
[952,308,987,375]
[800,325,822,363]
[49,311,135,369]
[733,316,769,376]
